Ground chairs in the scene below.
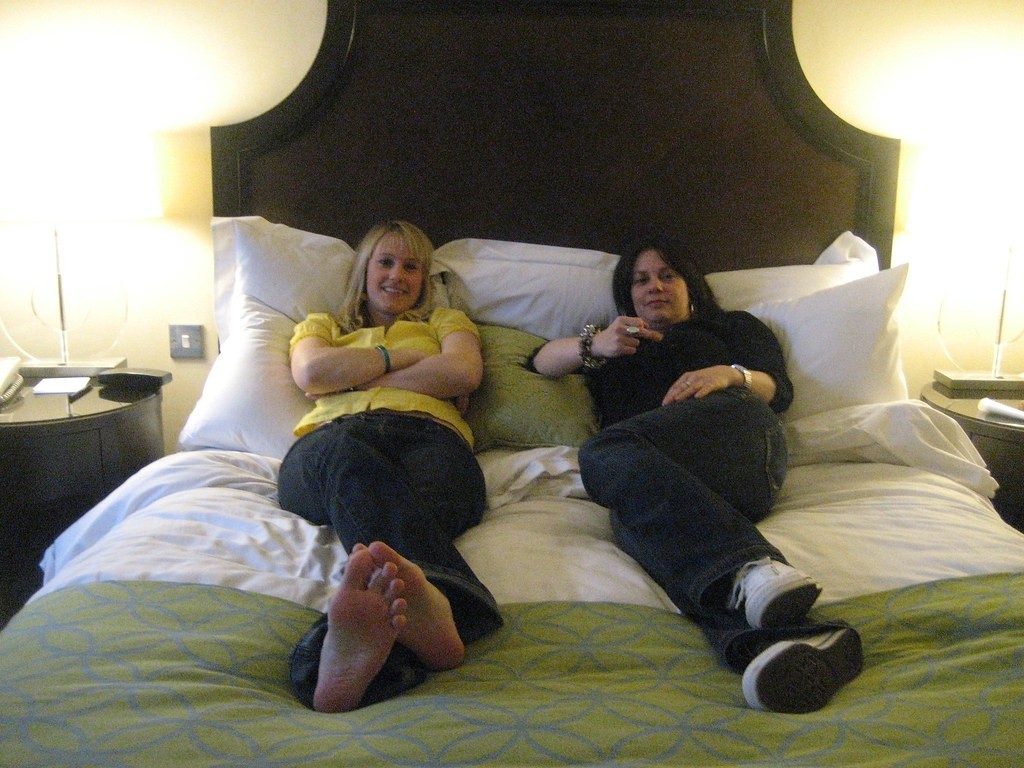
[0,0,1024,768]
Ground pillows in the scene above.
[460,321,602,457]
[211,213,451,322]
[739,261,911,421]
[432,239,624,341]
[702,230,881,307]
[180,293,317,463]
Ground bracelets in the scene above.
[580,324,608,371]
[376,343,391,373]
[349,387,355,392]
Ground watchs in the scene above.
[732,364,752,388]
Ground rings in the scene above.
[684,379,691,386]
[627,326,640,335]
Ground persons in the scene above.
[277,220,504,713]
[527,232,864,715]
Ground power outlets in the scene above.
[168,324,204,358]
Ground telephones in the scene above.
[0,355,25,403]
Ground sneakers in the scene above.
[741,621,865,713]
[732,559,823,628]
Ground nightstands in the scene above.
[921,382,1024,534]
[0,369,174,640]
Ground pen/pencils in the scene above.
[69,384,93,404]
